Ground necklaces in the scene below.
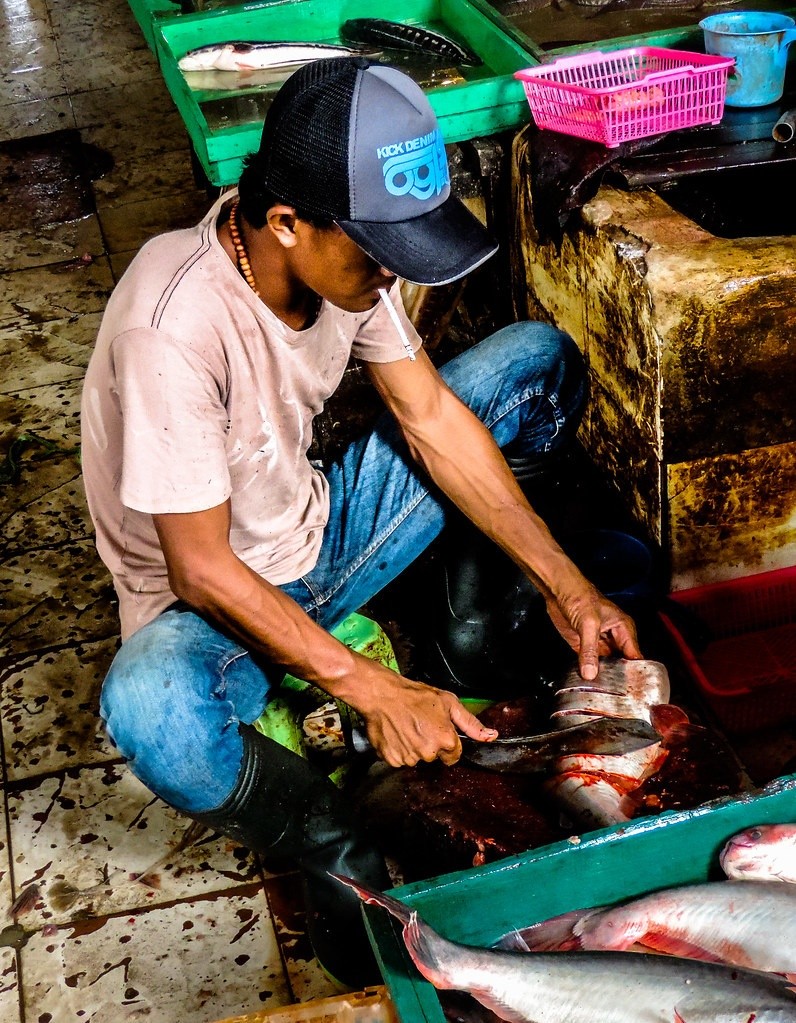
[228,201,260,296]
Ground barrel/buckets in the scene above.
[698,10,796,108]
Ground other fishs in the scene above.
[325,658,796,1022]
[173,0,665,121]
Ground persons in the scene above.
[79,57,642,989]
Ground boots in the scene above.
[157,722,403,987]
[410,449,572,705]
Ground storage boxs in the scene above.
[356,774,796,1023]
[129,0,703,188]
[658,566,796,733]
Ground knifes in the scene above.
[350,716,665,771]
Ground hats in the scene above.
[255,55,503,286]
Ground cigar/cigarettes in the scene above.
[377,289,416,361]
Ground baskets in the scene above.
[515,45,736,148]
